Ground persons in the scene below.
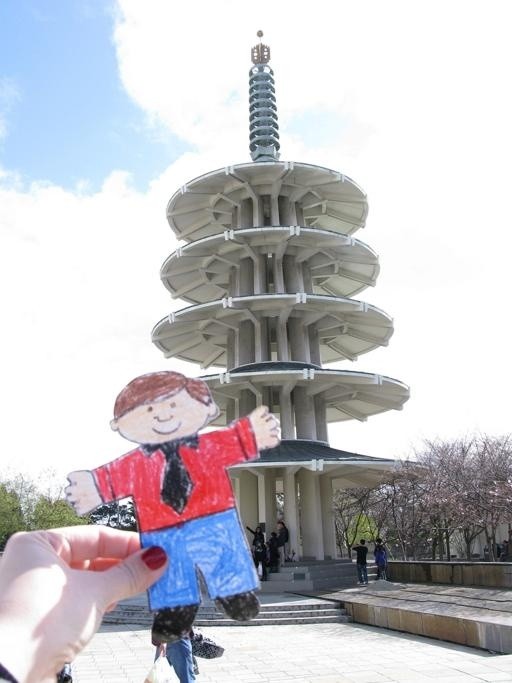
[374,538,390,581]
[154,628,199,683]
[352,539,368,584]
[246,521,295,581]
[0,524,168,683]
[65,370,281,646]
